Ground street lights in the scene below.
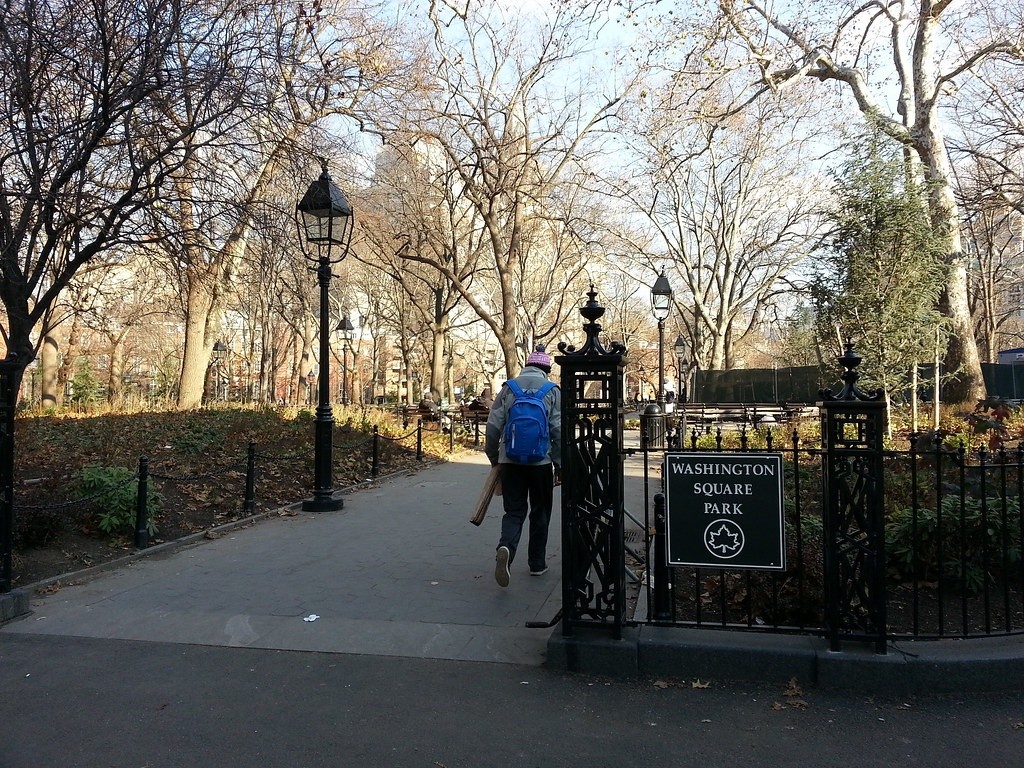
[307,370,314,408]
[461,375,468,399]
[649,265,674,414]
[211,339,228,400]
[417,374,423,400]
[680,359,689,405]
[334,318,354,410]
[371,378,377,404]
[638,365,646,404]
[293,158,346,511]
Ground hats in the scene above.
[526,344,551,373]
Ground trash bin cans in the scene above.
[639,404,665,449]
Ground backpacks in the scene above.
[503,379,560,464]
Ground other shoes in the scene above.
[530,565,549,575]
[495,546,511,586]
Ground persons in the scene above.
[484,343,560,587]
[419,392,451,434]
[481,388,496,423]
[468,395,482,431]
